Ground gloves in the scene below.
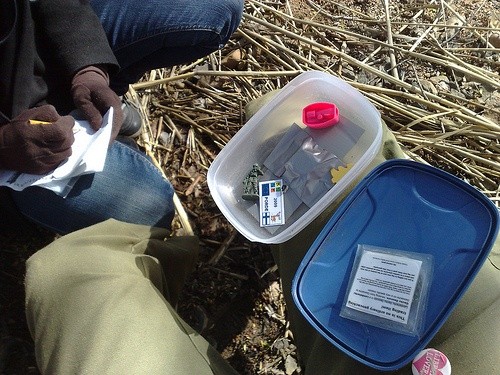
[0,105,74,175]
[70,66,123,147]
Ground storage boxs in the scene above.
[207,70,383,245]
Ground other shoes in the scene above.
[114,137,140,153]
[118,96,144,138]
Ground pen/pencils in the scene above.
[25,119,52,126]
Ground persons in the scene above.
[242,163,264,200]
[0,0,246,240]
[21,87,500,375]
